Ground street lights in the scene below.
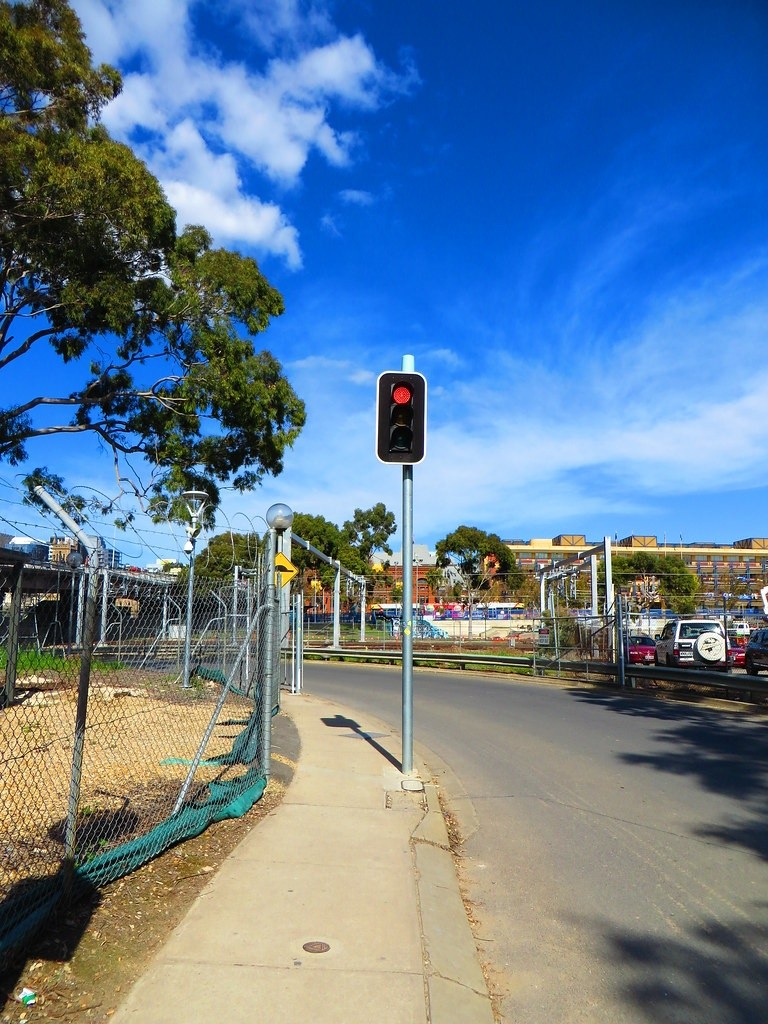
[175,488,211,689]
[413,558,424,619]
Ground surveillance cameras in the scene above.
[183,541,193,554]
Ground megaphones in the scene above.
[185,527,200,538]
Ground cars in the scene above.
[743,626,768,675]
[608,635,657,665]
[727,620,752,669]
[440,610,485,620]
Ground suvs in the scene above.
[654,619,734,672]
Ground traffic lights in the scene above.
[376,370,428,465]
